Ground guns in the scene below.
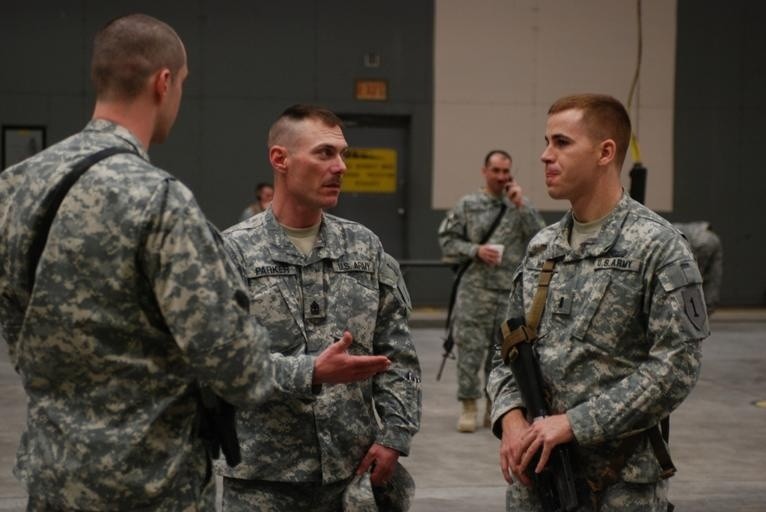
[494,316,599,512]
[205,395,242,468]
[436,316,455,382]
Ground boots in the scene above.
[484,400,491,427]
[456,398,478,432]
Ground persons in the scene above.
[239,183,274,224]
[222,106,422,512]
[1,14,391,510]
[438,152,545,434]
[485,95,712,511]
[673,222,722,317]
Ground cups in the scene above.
[485,246,506,265]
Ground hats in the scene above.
[342,460,416,512]
